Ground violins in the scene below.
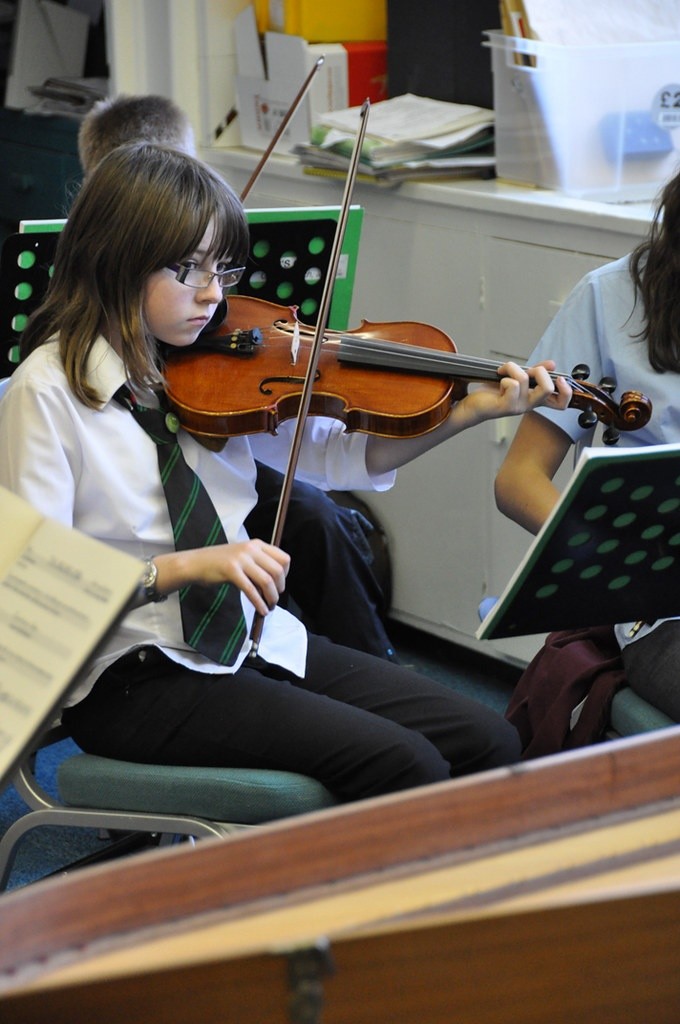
[163,295,653,445]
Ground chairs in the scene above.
[0,728,329,888]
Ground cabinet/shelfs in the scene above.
[214,164,647,669]
[0,109,83,239]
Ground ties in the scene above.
[114,383,249,668]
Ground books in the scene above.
[293,92,501,189]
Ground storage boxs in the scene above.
[481,30,680,204]
[233,0,387,159]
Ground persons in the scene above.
[0,145,574,805]
[493,171,680,726]
[77,93,401,664]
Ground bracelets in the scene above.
[143,555,168,603]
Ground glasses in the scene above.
[166,261,246,289]
